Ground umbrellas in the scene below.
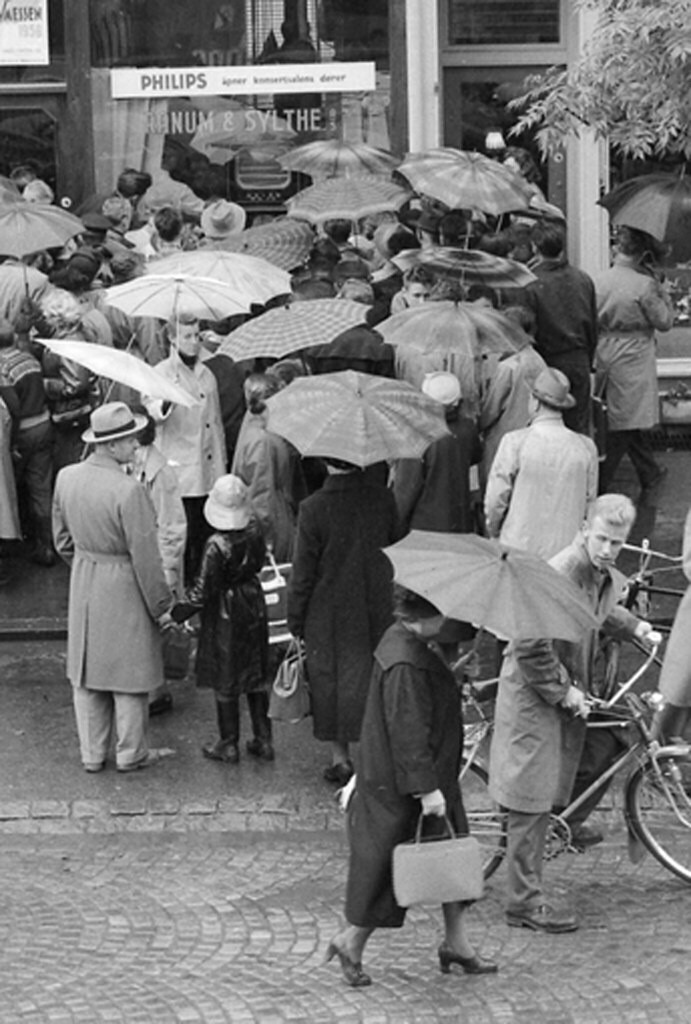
[372,298,534,357]
[213,297,374,363]
[214,221,316,271]
[399,145,538,213]
[276,139,399,180]
[262,369,452,464]
[596,172,691,241]
[189,110,299,166]
[389,246,538,288]
[283,174,410,222]
[0,204,86,258]
[32,338,199,411]
[107,272,253,320]
[145,251,294,306]
[379,530,602,641]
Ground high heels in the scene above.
[325,759,353,786]
[438,941,498,974]
[324,935,371,986]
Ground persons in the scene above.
[487,494,653,933]
[0,140,691,778]
[322,592,499,986]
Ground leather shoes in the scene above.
[507,903,580,932]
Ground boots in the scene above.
[29,516,55,566]
[203,699,240,762]
[247,690,273,760]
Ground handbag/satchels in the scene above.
[390,813,483,907]
[267,636,313,724]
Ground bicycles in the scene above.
[331,627,691,908]
[591,537,691,702]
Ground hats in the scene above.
[407,210,439,233]
[524,368,577,409]
[203,474,253,531]
[422,371,463,412]
[82,212,112,233]
[201,200,246,238]
[81,401,149,443]
[394,589,440,619]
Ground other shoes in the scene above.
[568,825,604,845]
[117,748,175,771]
[149,692,173,714]
[83,762,104,772]
[644,466,667,489]
